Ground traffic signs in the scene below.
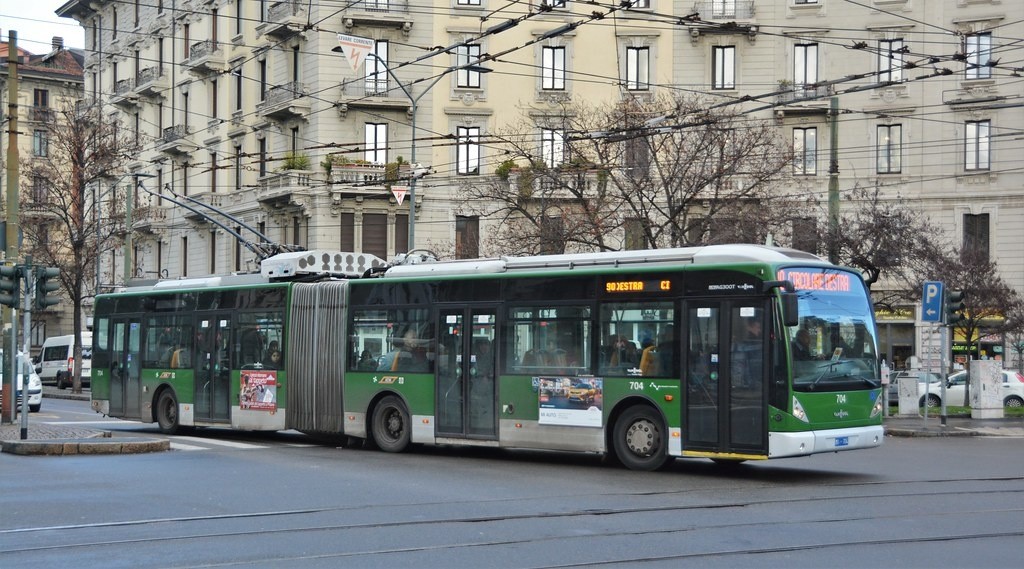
[921,281,943,322]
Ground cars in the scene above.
[0,347,43,412]
[886,369,941,406]
[917,367,1024,410]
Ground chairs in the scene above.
[171,349,187,368]
[524,346,659,377]
[392,351,400,372]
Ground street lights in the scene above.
[331,45,417,256]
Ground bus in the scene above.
[88,180,898,476]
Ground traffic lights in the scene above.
[0,264,21,309]
[945,287,966,325]
[35,265,60,310]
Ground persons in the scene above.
[159,326,496,391]
[743,317,761,346]
[790,330,817,361]
[623,323,676,377]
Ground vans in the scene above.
[32,331,101,390]
